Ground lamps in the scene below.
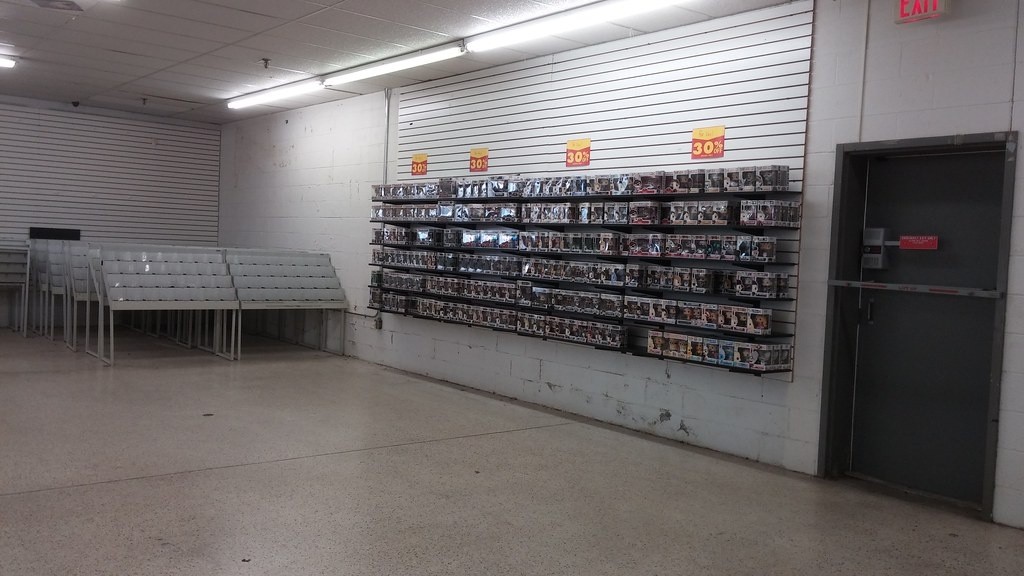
[226,0,671,110]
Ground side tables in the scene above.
[367,164,801,377]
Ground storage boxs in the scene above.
[368,165,801,378]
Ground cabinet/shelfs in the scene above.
[367,187,801,375]
